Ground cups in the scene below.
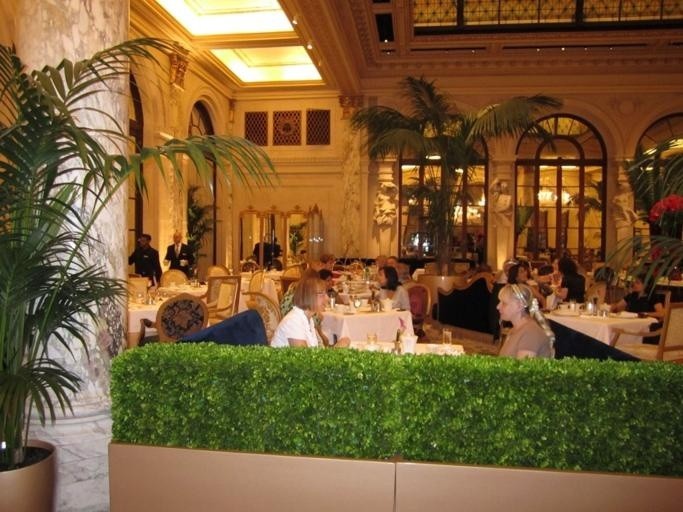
[442,328,452,349]
[400,335,418,355]
[135,277,207,304]
[344,258,374,282]
[367,332,377,344]
[557,299,608,317]
[328,291,391,312]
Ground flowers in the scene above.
[646,191,682,240]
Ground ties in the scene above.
[175,244,179,258]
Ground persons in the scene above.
[372,180,397,227]
[497,257,665,357]
[488,177,512,226]
[272,257,408,349]
[251,241,272,268]
[162,232,194,276]
[127,233,160,287]
[272,237,280,258]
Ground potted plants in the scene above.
[0,34,281,512]
